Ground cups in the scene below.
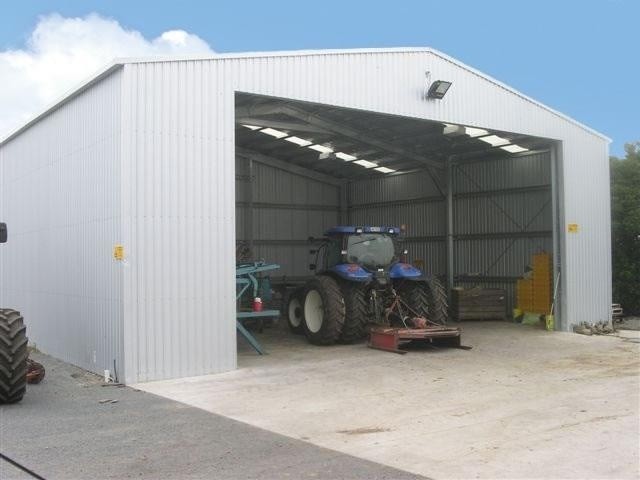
[253,297,263,311]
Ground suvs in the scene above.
[612,304,623,322]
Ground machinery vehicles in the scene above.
[288,225,449,345]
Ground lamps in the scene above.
[428,80,452,100]
